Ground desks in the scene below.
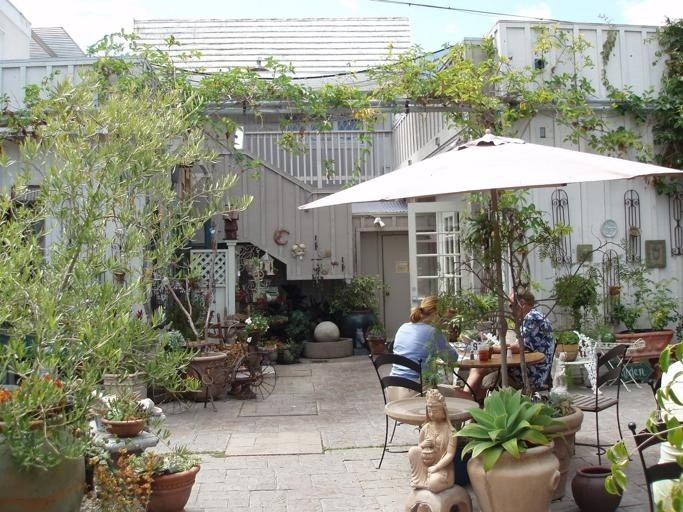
[592,336,647,394]
[434,348,547,406]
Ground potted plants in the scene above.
[327,270,392,354]
[519,387,585,502]
[553,327,581,362]
[449,386,563,512]
[0,21,304,511]
[608,301,676,357]
[363,322,388,355]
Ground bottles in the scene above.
[507,347,513,358]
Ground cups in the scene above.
[478,344,490,361]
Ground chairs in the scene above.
[625,418,682,512]
[562,342,634,468]
[520,342,559,401]
[365,349,432,471]
[550,330,602,395]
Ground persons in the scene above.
[481,290,554,389]
[387,296,481,400]
[409,388,458,493]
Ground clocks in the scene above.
[599,217,619,240]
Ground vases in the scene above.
[569,464,625,512]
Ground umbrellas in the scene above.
[297,128,683,389]
